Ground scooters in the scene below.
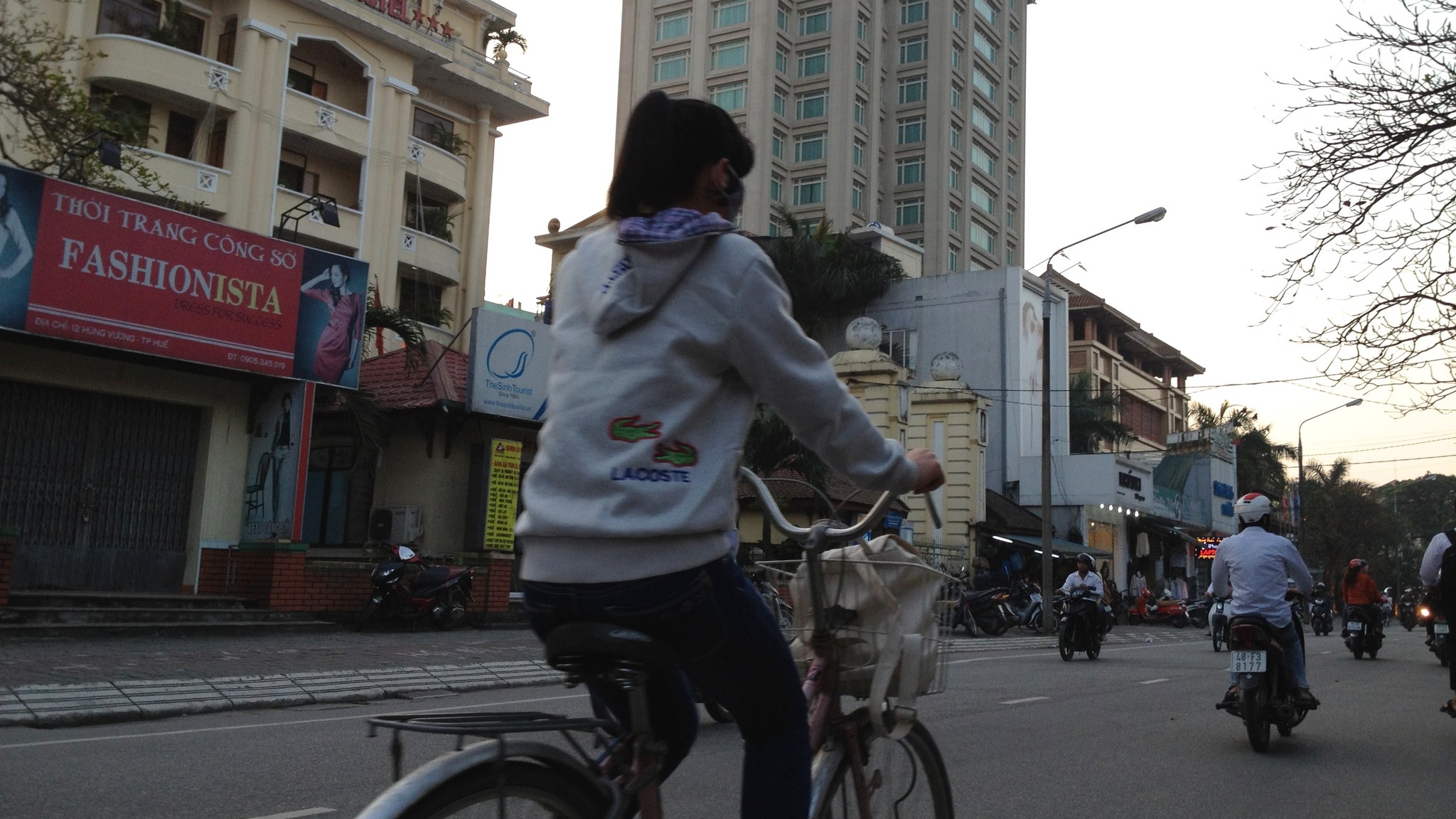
[935,565,1456,668]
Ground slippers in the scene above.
[1440,700,1456,719]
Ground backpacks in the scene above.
[788,519,946,740]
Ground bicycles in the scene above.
[355,464,961,819]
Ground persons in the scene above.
[1129,571,1147,598]
[506,85,951,819]
[299,259,366,387]
[1312,557,1447,654]
[1210,492,1322,708]
[1418,518,1456,718]
[1055,553,1113,643]
[1,172,33,280]
[264,390,297,541]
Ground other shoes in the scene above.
[1340,627,1349,638]
[1223,692,1237,703]
[1296,694,1321,706]
[1374,632,1385,639]
[1424,640,1433,645]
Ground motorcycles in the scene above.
[1206,582,1324,758]
[351,539,480,632]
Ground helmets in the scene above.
[1078,553,1092,563]
[1234,493,1272,523]
[1316,582,1325,589]
[1348,559,1365,568]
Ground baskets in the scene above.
[755,554,965,699]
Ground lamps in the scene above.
[318,200,340,228]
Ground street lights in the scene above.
[1039,205,1170,635]
[1298,398,1363,561]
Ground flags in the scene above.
[1279,489,1302,529]
